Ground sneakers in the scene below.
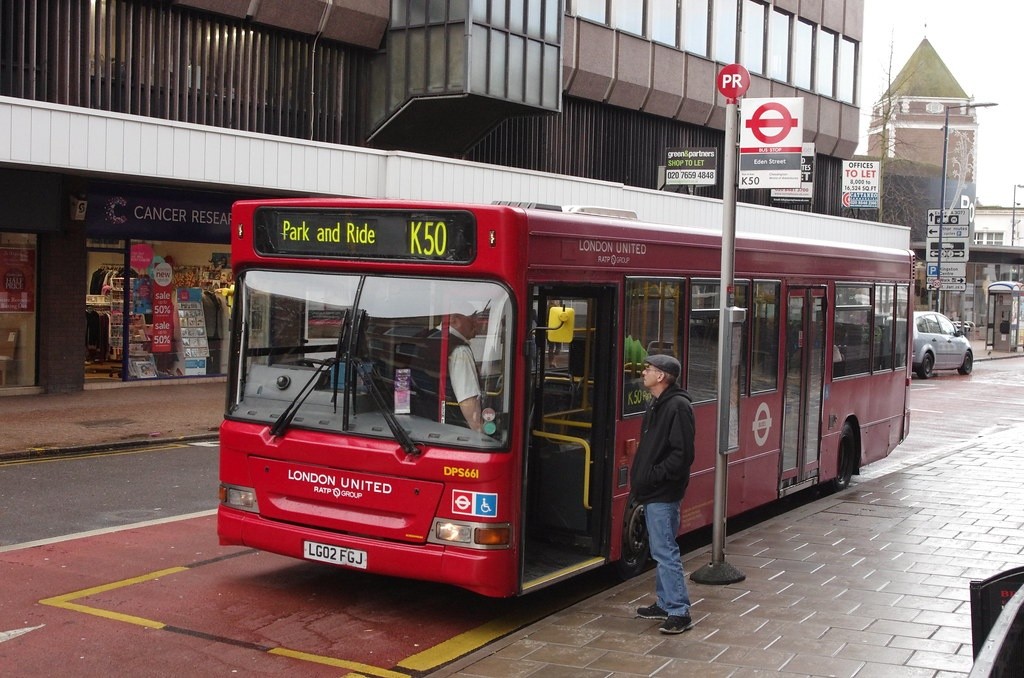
[657,612,693,634]
[636,603,668,620]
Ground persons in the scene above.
[630,354,695,634]
[411,304,489,435]
[547,300,562,368]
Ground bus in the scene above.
[216,199,916,599]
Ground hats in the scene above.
[646,354,680,378]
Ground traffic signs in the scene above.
[927,207,970,291]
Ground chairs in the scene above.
[490,319,893,436]
[0,328,20,385]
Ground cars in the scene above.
[910,311,973,377]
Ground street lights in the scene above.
[935,102,1000,313]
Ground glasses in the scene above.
[647,367,663,373]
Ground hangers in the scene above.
[85,264,133,316]
